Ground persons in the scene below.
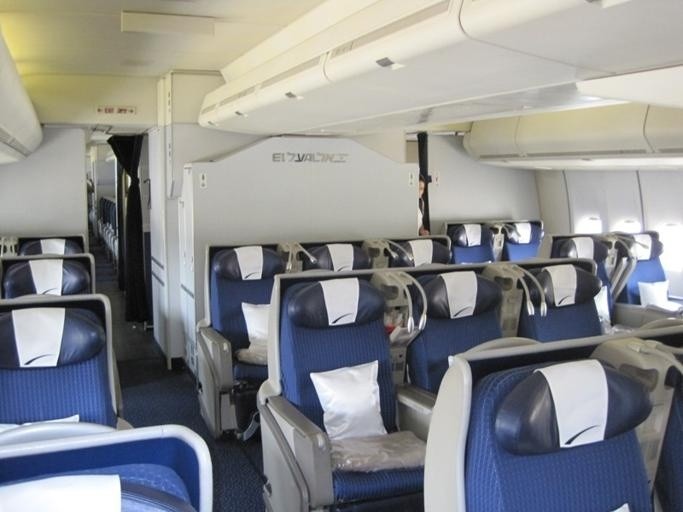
[418,172,431,236]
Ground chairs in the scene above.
[0,423,214,512]
[0,233,89,257]
[255,266,441,512]
[625,315,682,512]
[0,253,97,302]
[0,292,135,447]
[490,218,545,261]
[194,243,296,441]
[398,260,535,399]
[423,336,683,512]
[297,239,374,272]
[548,230,683,334]
[505,255,612,344]
[377,233,453,269]
[443,220,498,265]
